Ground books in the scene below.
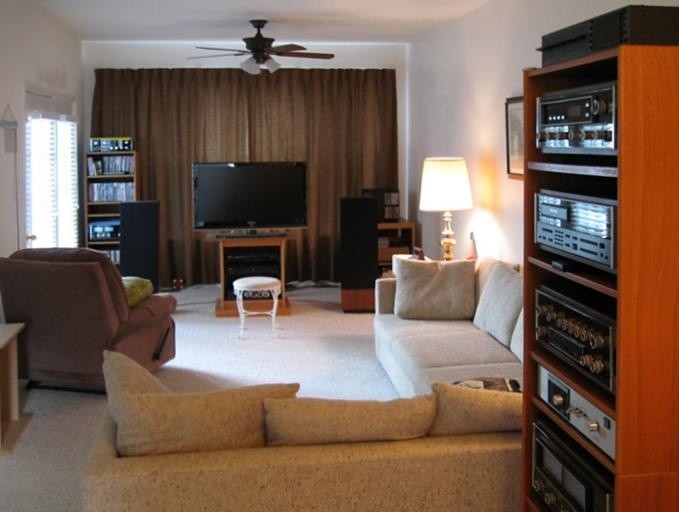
[101,248,120,263]
[87,155,134,176]
[87,181,134,202]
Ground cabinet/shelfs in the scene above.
[82,150,141,274]
[1,323,33,454]
[361,188,401,222]
[520,45,679,511]
[378,216,416,277]
[215,236,292,316]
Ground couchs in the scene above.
[1,248,177,394]
[374,257,524,400]
[82,399,523,511]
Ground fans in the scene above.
[186,19,335,60]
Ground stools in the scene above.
[232,276,283,340]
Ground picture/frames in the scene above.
[505,95,524,180]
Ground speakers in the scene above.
[339,195,378,290]
[119,200,161,294]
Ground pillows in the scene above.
[427,382,524,437]
[103,348,174,422]
[116,383,302,456]
[260,394,438,446]
[121,275,154,308]
[393,256,476,320]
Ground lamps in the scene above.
[419,157,474,261]
[241,58,263,76]
[263,57,280,75]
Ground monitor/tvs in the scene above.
[190,160,309,237]
